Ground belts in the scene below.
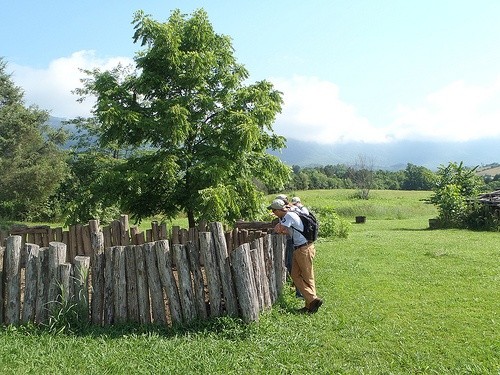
[294,242,311,250]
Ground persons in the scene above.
[274,194,310,299]
[267,199,323,313]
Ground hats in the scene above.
[275,194,288,201]
[267,199,285,210]
[290,197,301,205]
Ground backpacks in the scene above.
[287,211,317,242]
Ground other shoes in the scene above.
[308,298,323,313]
[300,307,310,312]
